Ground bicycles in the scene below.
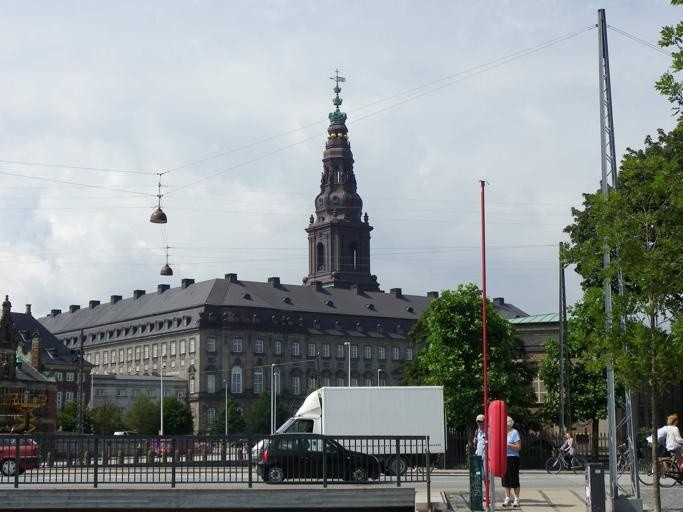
[617,436,683,488]
[545,447,588,474]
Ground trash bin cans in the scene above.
[584,463,605,512]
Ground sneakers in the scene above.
[503,498,511,506]
[513,500,520,507]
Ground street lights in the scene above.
[344,341,351,388]
[160,352,166,437]
[270,363,276,436]
[222,379,228,436]
[377,368,382,385]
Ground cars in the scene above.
[231,441,244,448]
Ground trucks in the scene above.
[250,384,447,476]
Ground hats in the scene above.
[476,414,485,422]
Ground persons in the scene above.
[472,413,495,503]
[561,433,577,470]
[645,414,683,471]
[502,416,521,507]
[617,433,642,467]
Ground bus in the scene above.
[151,438,194,457]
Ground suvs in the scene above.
[0,433,40,477]
[256,432,381,483]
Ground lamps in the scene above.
[147,175,173,277]
